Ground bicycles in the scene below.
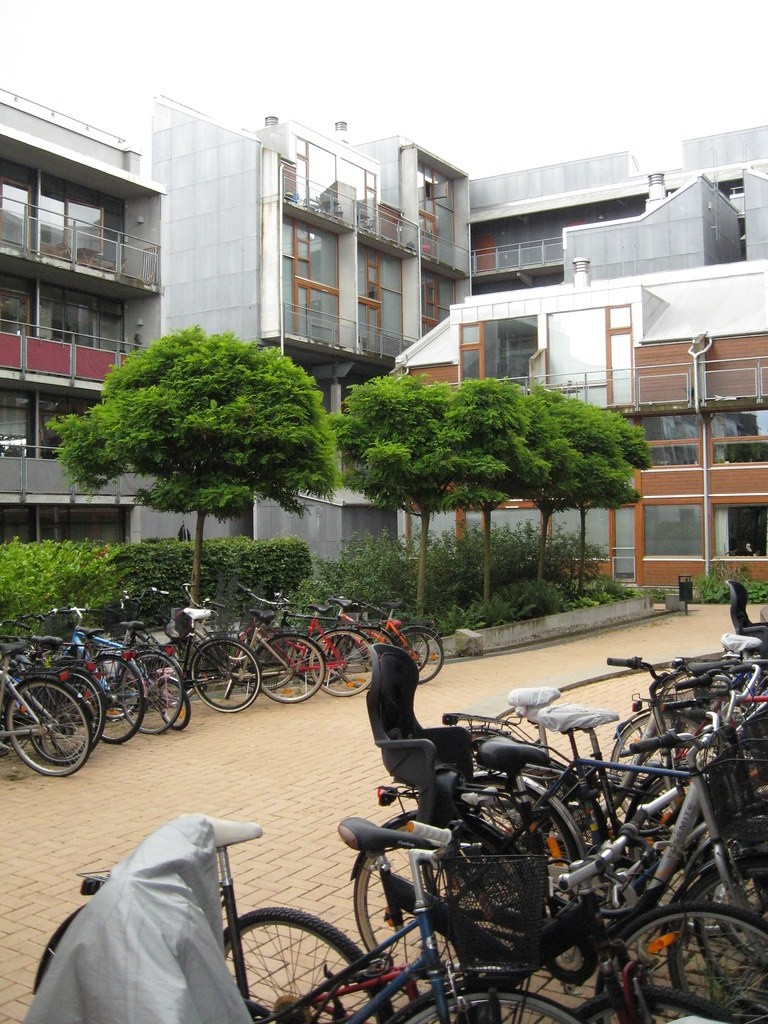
[0,581,768,1024]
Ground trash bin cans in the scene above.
[678,574,693,601]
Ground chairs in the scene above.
[726,579,768,657]
[56,240,71,260]
[363,643,475,831]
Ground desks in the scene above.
[76,247,100,268]
